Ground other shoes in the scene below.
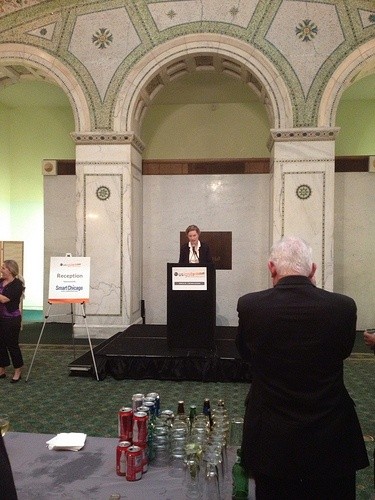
[9,374,21,383]
[0,374,6,379]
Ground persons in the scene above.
[0,260,26,383]
[235,238,369,500]
[179,225,211,264]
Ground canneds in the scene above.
[116,441,142,481]
[118,393,160,473]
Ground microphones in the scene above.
[192,245,195,253]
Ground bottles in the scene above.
[232,449,249,500]
[182,461,220,500]
[177,399,225,432]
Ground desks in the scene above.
[0,432,256,500]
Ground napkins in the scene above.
[46,432,88,451]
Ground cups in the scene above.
[150,410,228,487]
[0,414,10,438]
